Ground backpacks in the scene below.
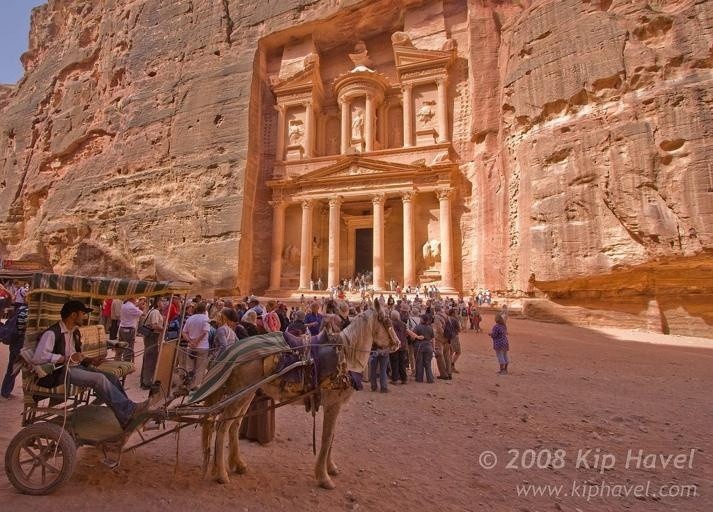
[438,313,456,340]
[164,312,184,340]
[338,314,350,331]
[0,306,28,345]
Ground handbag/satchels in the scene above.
[206,355,218,373]
[137,325,153,338]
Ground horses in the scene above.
[201,297,402,489]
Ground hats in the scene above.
[59,299,95,319]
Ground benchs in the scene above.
[25,324,136,405]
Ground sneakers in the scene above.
[121,395,152,433]
[140,382,151,390]
[436,374,452,380]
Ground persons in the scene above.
[349,110,363,138]
[0,266,509,401]
[30,300,153,433]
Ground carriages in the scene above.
[5,273,401,496]
